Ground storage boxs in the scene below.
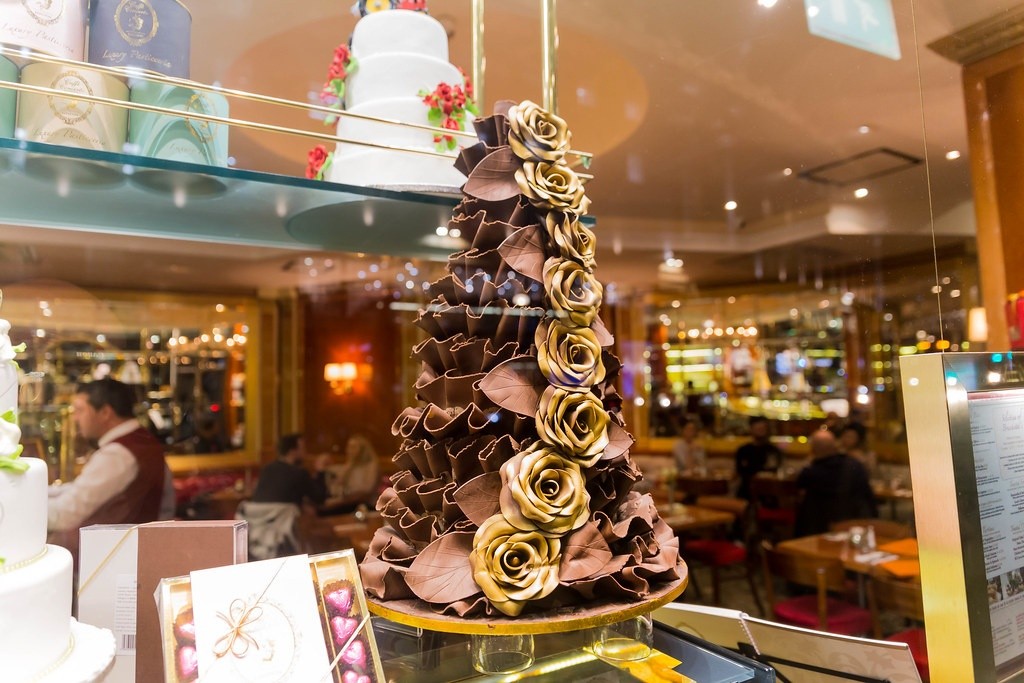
[77,520,248,683]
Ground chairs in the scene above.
[759,538,878,640]
[678,496,765,616]
[864,574,932,683]
[748,472,797,542]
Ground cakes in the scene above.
[0,317,73,683]
[318,9,482,187]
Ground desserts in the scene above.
[175,588,372,683]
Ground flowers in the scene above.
[403,100,632,618]
[303,0,478,188]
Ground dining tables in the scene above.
[632,455,926,640]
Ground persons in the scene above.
[319,435,378,518]
[253,434,331,508]
[665,382,880,602]
[46,377,179,589]
